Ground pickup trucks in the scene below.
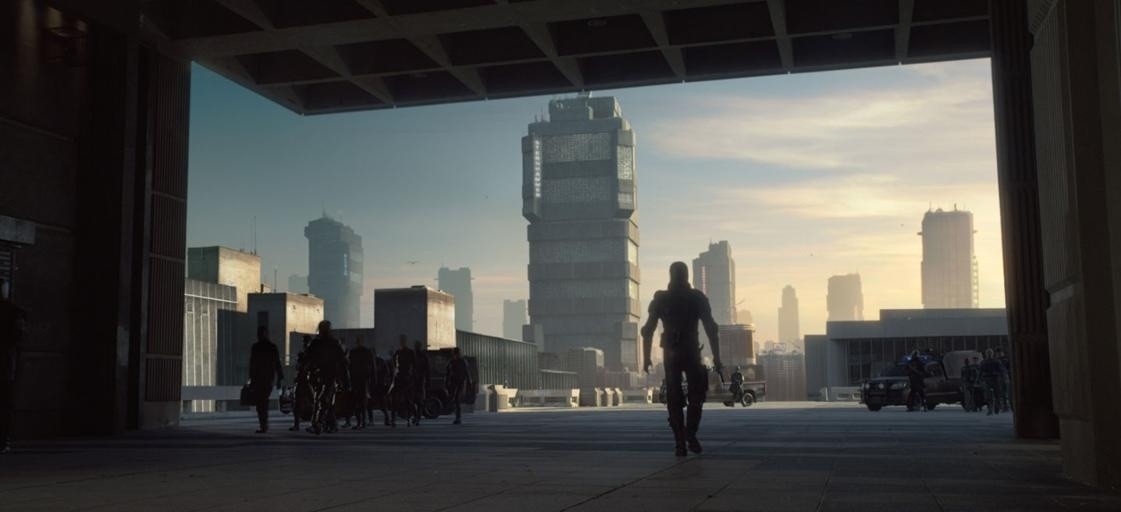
[659,366,767,407]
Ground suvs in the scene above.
[858,349,982,413]
[278,347,479,422]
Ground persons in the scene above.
[250,322,474,435]
[642,261,722,455]
[906,350,929,412]
[959,346,1014,414]
[730,365,744,407]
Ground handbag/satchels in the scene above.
[241,381,257,405]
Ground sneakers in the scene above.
[668,435,704,456]
[255,415,465,434]
